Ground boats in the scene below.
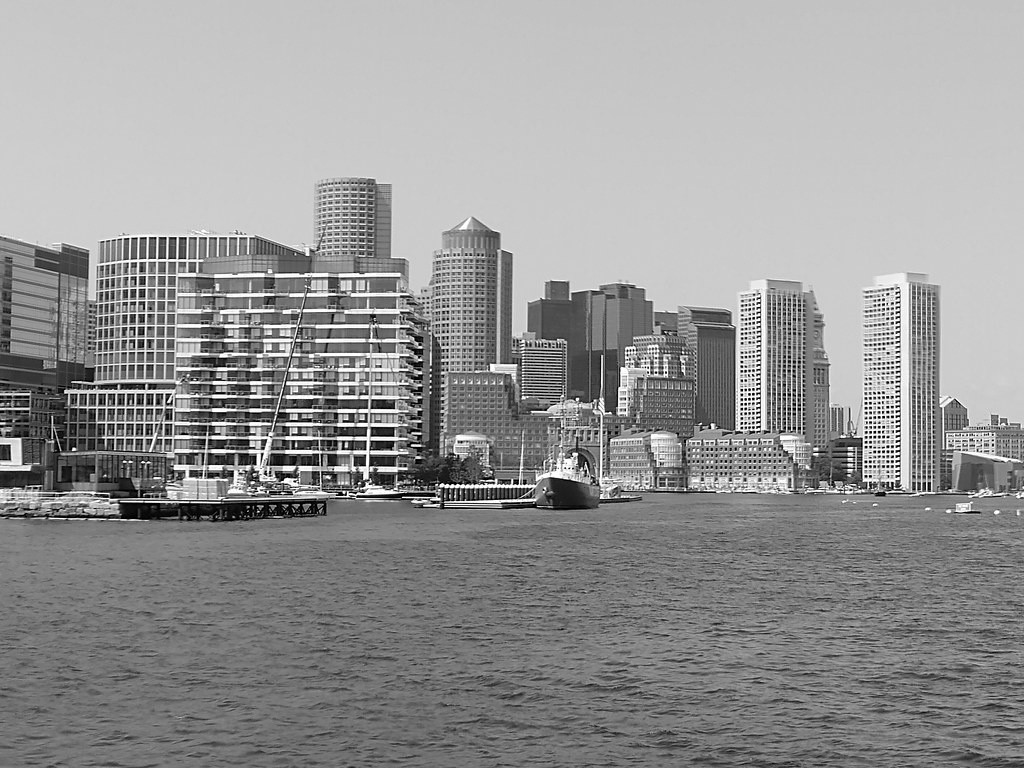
[226,280,315,497]
[534,387,602,508]
[873,473,887,497]
